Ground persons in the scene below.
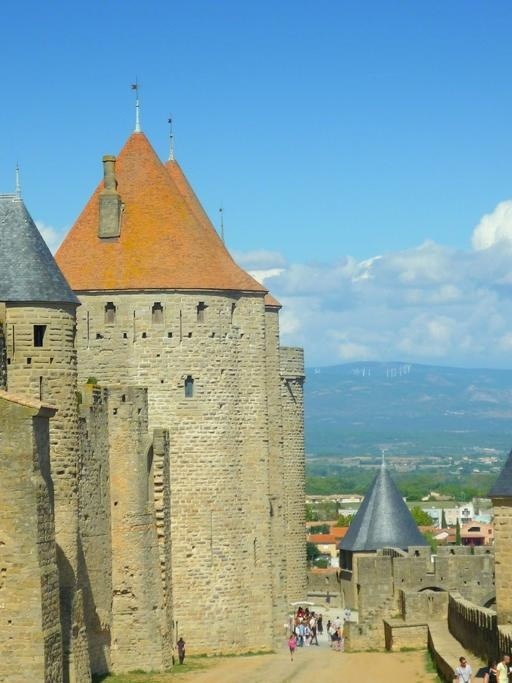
[326,590,330,604]
[287,606,351,660]
[483,657,497,683]
[177,637,186,665]
[495,655,512,682]
[454,656,473,683]
